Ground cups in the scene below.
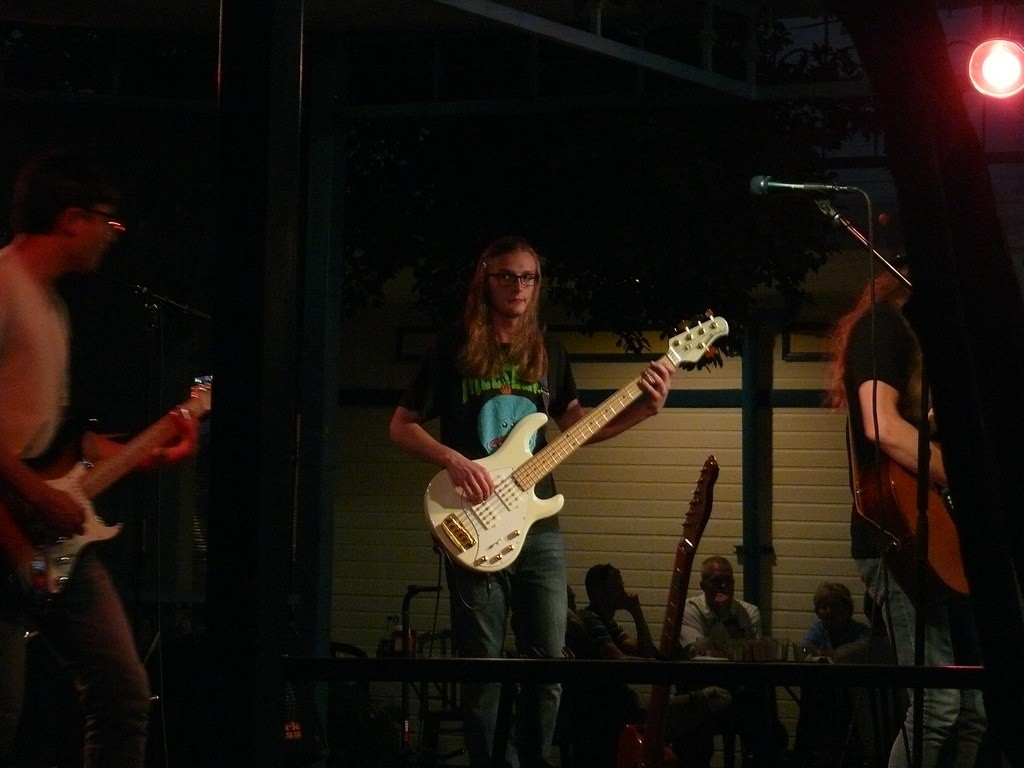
[794,645,809,662]
[696,637,713,656]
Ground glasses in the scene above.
[82,206,125,237]
[486,272,540,287]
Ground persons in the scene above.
[388,233,676,768]
[562,556,879,768]
[829,192,984,768]
[0,155,200,768]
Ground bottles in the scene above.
[384,616,394,639]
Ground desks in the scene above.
[269,655,984,768]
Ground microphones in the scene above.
[751,175,860,195]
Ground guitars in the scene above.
[618,454,719,768]
[424,308,730,574]
[0,373,212,616]
[856,439,969,597]
